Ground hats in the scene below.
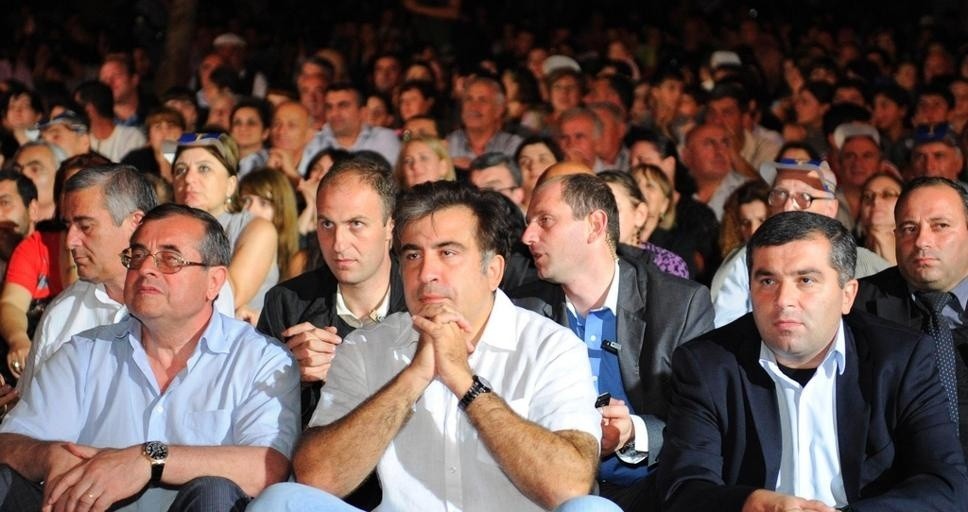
[164,132,239,172]
[834,122,881,150]
[542,55,582,76]
[711,51,742,70]
[213,33,247,45]
[904,122,959,150]
[29,104,90,131]
[759,158,836,195]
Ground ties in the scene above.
[913,290,968,449]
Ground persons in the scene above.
[2,0,968,512]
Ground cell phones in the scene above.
[595,393,611,411]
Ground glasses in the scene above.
[117,246,206,274]
[861,189,900,202]
[766,188,834,208]
